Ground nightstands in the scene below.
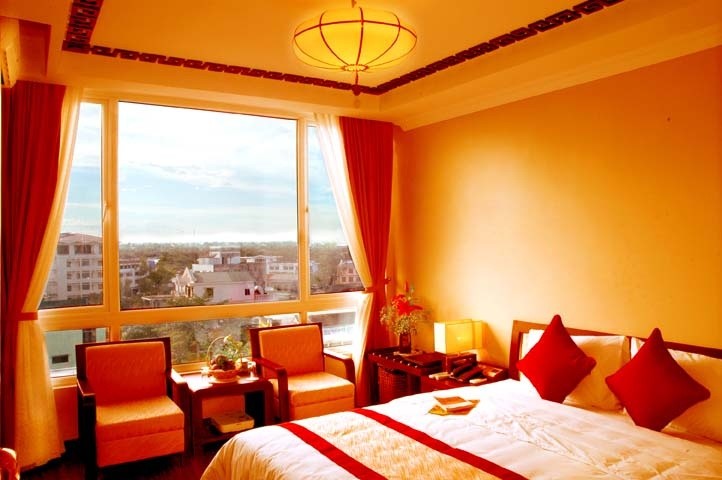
[419,374,488,393]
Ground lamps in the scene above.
[434,319,485,373]
[292,0,419,74]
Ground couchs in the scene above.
[75,336,191,480]
[248,322,357,422]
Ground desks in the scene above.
[366,345,442,402]
[183,370,275,457]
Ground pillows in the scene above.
[623,336,722,444]
[605,327,712,432]
[520,327,630,411]
[515,314,599,404]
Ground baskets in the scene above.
[202,337,243,384]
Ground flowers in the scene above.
[378,278,432,335]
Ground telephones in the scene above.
[448,363,482,382]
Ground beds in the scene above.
[198,319,722,480]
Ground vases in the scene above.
[398,331,411,354]
